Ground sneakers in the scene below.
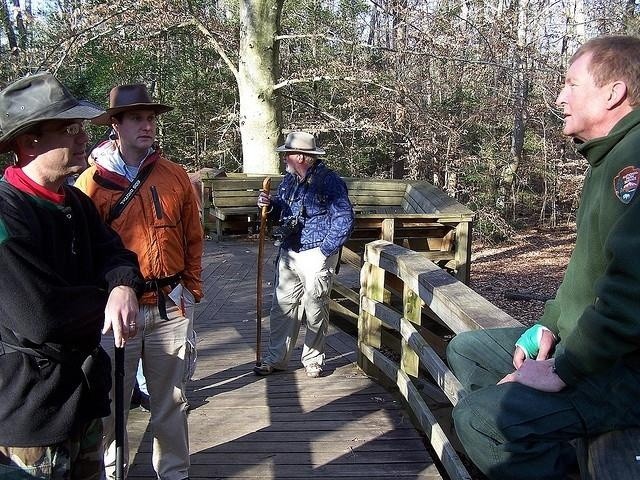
[305,363,322,377]
[254,362,274,374]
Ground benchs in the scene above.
[202,179,476,305]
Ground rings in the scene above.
[129,323,136,326]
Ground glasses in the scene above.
[43,120,87,135]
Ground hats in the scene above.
[276,132,326,154]
[90,84,174,127]
[0,72,106,153]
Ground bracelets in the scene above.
[552,362,568,386]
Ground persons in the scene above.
[250,129,355,380]
[444,31,639,479]
[0,70,150,480]
[87,138,163,413]
[74,82,206,480]
